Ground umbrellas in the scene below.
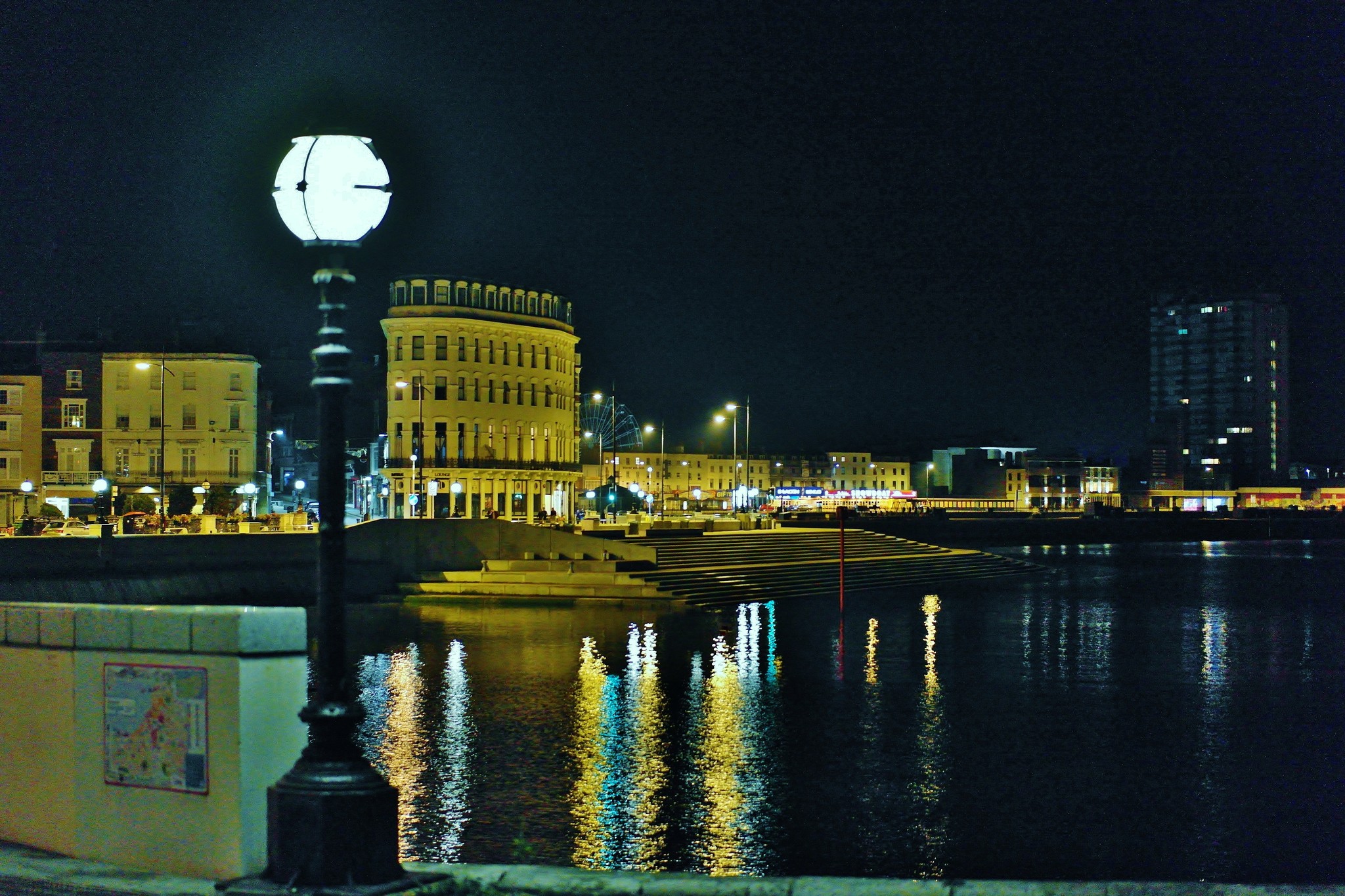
[121,511,147,531]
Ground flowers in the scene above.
[144,512,279,529]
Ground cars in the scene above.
[787,504,814,511]
[305,500,319,529]
[759,505,774,512]
[40,519,89,535]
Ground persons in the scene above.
[440,507,586,527]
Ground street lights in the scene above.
[870,464,878,511]
[715,394,757,514]
[645,418,669,520]
[265,430,283,474]
[833,463,839,490]
[682,462,690,515]
[395,368,431,519]
[590,380,617,524]
[20,476,31,518]
[136,346,174,535]
[203,478,213,515]
[214,130,452,896]
[94,472,107,524]
[451,479,462,517]
[926,463,933,506]
[243,477,255,521]
[776,463,784,486]
[295,477,304,511]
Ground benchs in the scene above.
[582,530,625,538]
[645,527,704,537]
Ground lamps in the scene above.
[209,421,215,425]
